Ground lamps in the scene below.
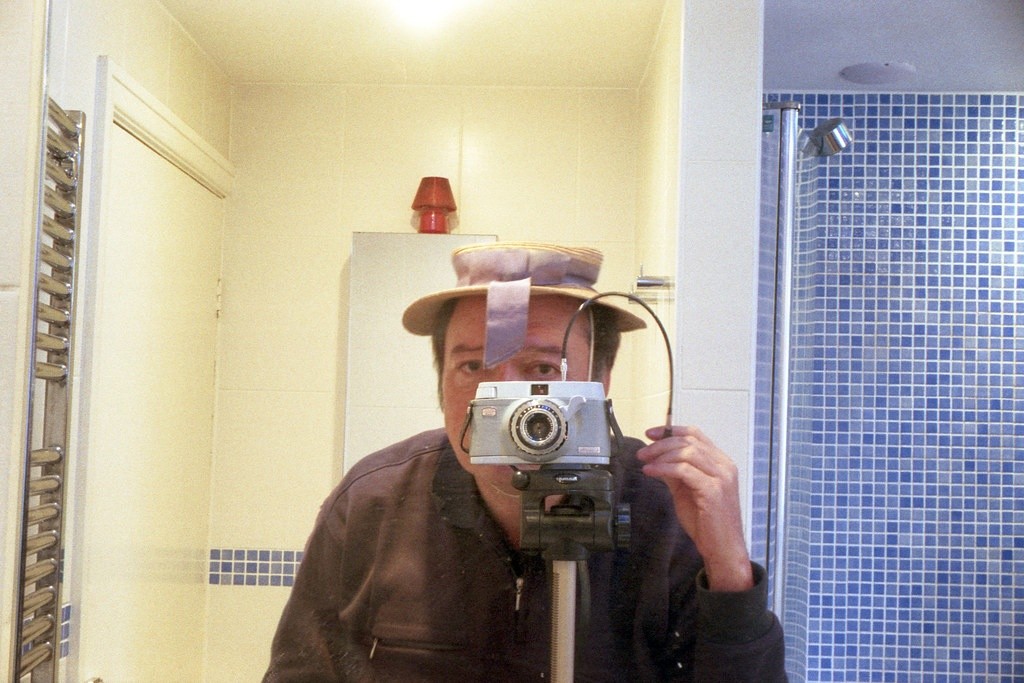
[412,177,457,234]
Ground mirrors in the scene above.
[14,0,1024,683]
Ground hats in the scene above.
[403,245,646,371]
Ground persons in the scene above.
[257,242,787,682]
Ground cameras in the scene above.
[469,381,610,465]
